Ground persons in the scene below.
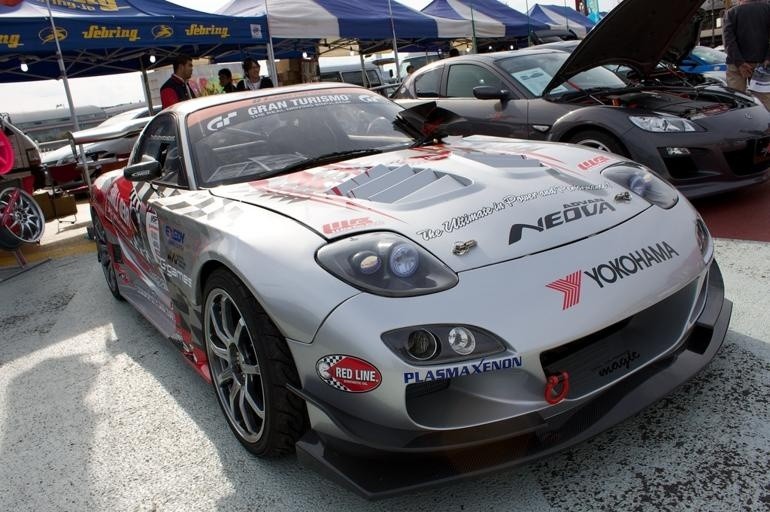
[237,58,274,91]
[721,0,770,92]
[448,49,459,57]
[218,68,237,94]
[160,52,196,109]
[401,65,415,82]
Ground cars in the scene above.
[0,103,165,191]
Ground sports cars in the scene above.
[87,79,734,501]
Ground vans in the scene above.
[318,49,443,94]
